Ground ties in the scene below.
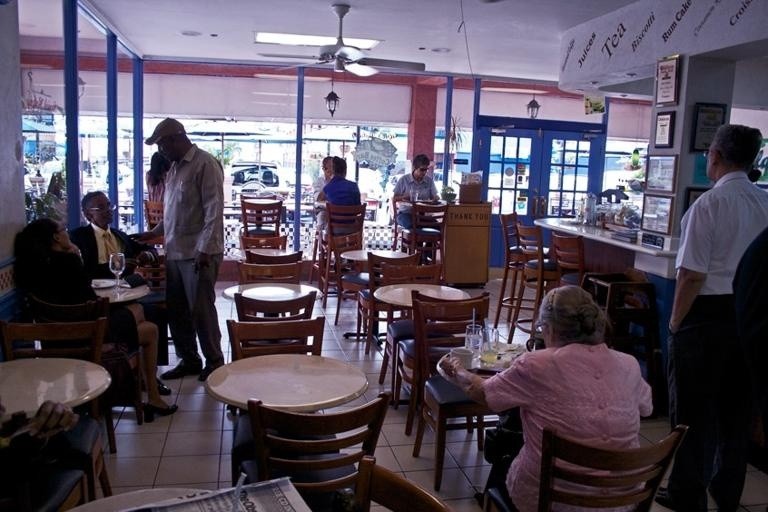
[104,232,114,261]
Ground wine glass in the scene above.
[108,252,126,292]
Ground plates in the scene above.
[90,280,115,288]
[416,199,433,202]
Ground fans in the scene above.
[252,4,429,80]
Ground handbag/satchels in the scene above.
[484,427,524,462]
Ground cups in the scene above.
[448,324,546,370]
[409,190,419,202]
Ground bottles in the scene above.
[577,193,641,229]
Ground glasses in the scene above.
[86,204,119,212]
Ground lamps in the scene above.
[332,59,346,74]
[322,76,343,119]
[526,84,542,121]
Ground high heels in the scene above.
[144,403,180,422]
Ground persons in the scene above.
[636,122,768,512]
[464,284,654,511]
[65,192,160,287]
[316,155,360,274]
[0,395,82,512]
[322,157,334,182]
[730,225,767,476]
[393,153,439,266]
[143,118,227,382]
[14,218,179,426]
[746,168,762,183]
[128,150,222,242]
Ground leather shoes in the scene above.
[159,360,227,380]
[655,485,708,511]
[145,380,173,395]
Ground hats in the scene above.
[146,117,187,146]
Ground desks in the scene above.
[204,353,370,414]
[69,488,211,511]
[221,282,325,317]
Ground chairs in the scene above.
[226,291,326,361]
[0,198,167,511]
[411,299,507,492]
[309,196,689,435]
[235,194,304,285]
[232,391,453,512]
[483,422,691,512]
